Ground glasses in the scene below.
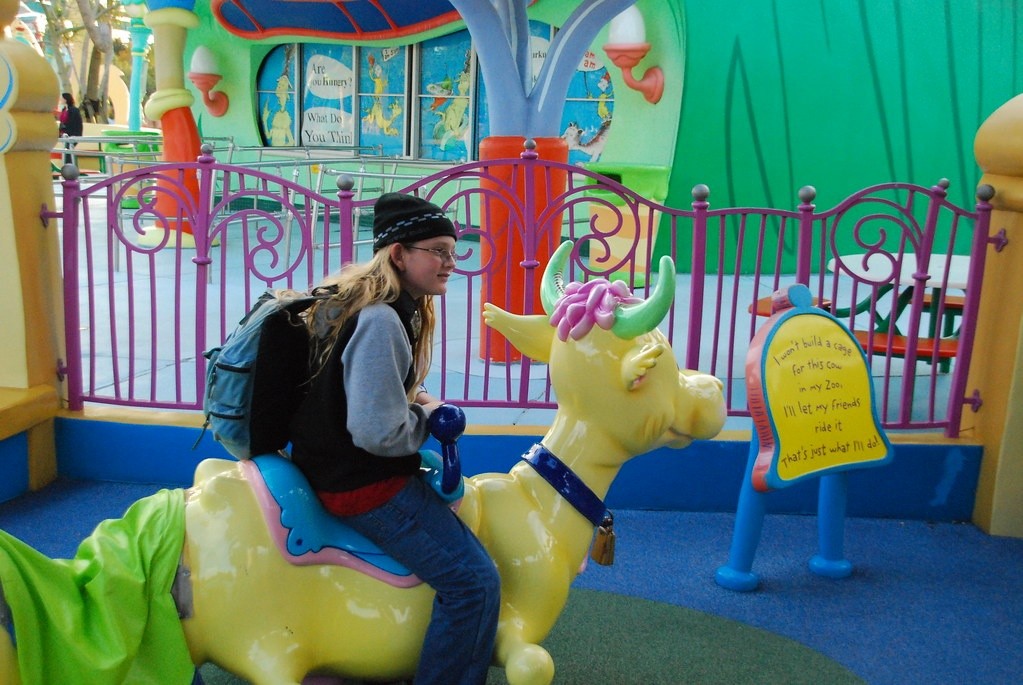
[402,244,458,263]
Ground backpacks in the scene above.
[202,290,344,461]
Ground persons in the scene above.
[57,93,83,165]
[292,192,501,685]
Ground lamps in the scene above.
[602,5,666,103]
[186,45,229,116]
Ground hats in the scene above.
[372,193,458,251]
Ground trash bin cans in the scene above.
[100,131,161,211]
[578,162,672,289]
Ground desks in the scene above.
[827,251,970,375]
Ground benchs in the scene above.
[749,293,965,356]
